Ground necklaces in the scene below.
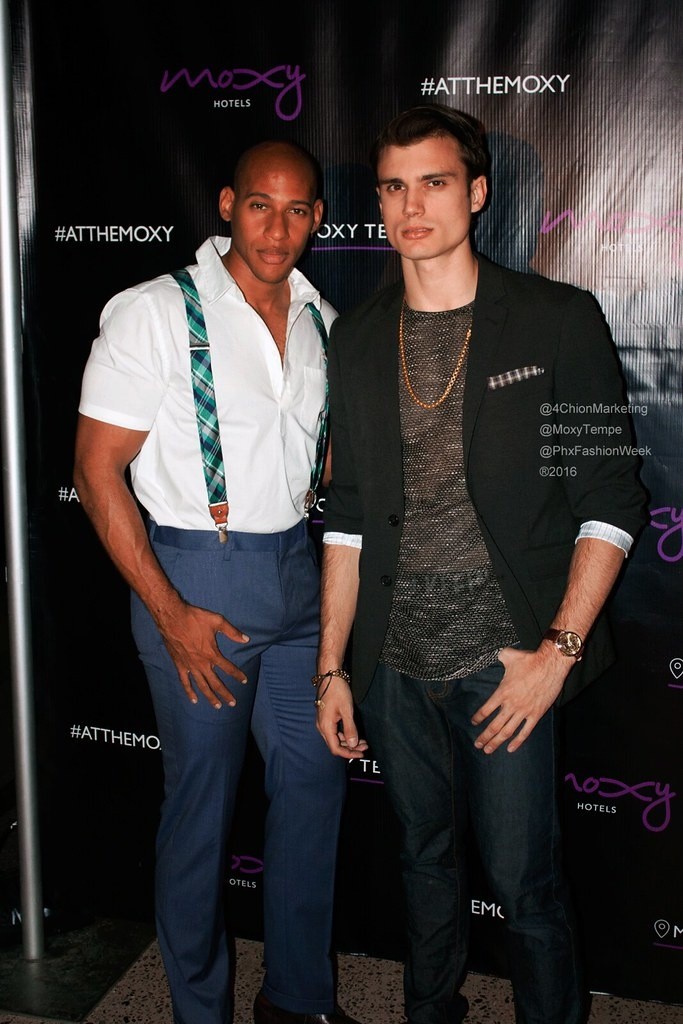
[399,289,471,408]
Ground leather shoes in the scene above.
[253,991,360,1024]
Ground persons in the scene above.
[314,103,652,1024]
[69,136,356,1024]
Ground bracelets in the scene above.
[311,669,351,708]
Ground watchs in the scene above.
[543,629,584,661]
